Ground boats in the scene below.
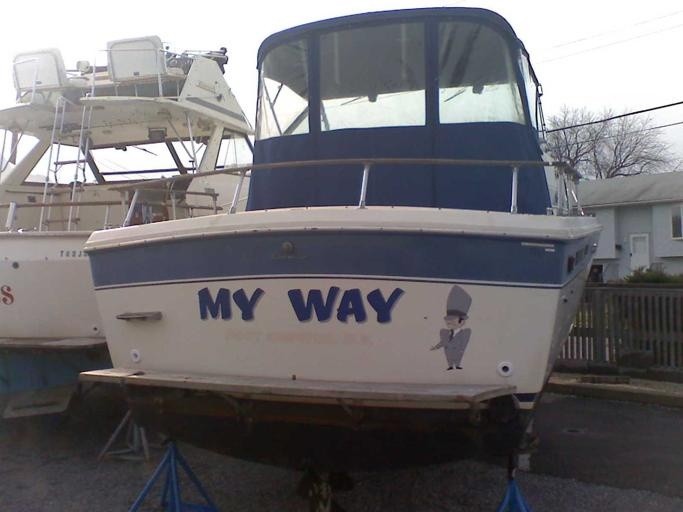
[0,32,254,422]
[77,7,604,480]
